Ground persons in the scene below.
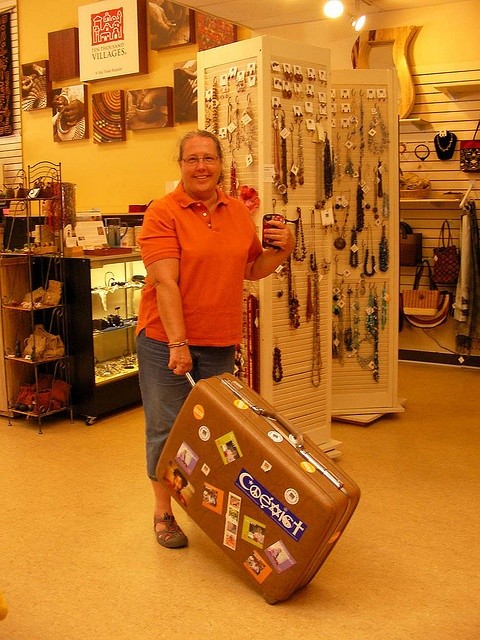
[124,86,173,130]
[135,131,296,549]
[20,61,47,111]
[51,85,85,142]
[148,0,190,48]
[174,60,197,122]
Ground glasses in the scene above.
[180,154,221,163]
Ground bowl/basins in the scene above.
[135,103,158,123]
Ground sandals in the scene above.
[154,512,188,548]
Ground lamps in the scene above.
[321,0,368,34]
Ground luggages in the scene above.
[154,372,361,605]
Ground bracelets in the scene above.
[167,339,188,348]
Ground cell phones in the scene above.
[261,213,285,251]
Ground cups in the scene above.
[103,217,128,249]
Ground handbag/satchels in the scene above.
[460,119,479,173]
[399,221,422,267]
[403,260,438,316]
[433,220,459,284]
[400,259,452,328]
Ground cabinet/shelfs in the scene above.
[28,251,155,423]
[0,160,76,434]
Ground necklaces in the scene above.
[366,290,373,332]
[436,131,454,154]
[351,289,360,348]
[358,95,365,162]
[367,106,390,155]
[378,221,388,272]
[235,95,241,149]
[242,100,254,151]
[273,347,283,382]
[337,291,345,364]
[332,328,338,359]
[282,116,288,204]
[333,262,340,316]
[336,138,342,177]
[290,129,297,189]
[293,206,307,261]
[310,210,317,272]
[306,273,311,323]
[227,97,232,143]
[332,204,350,250]
[315,141,326,210]
[212,76,219,135]
[377,161,383,197]
[356,167,365,231]
[344,149,355,176]
[373,175,378,213]
[288,276,301,330]
[321,224,334,270]
[364,226,376,276]
[311,273,321,388]
[356,338,375,368]
[298,124,304,185]
[229,161,237,197]
[323,139,334,198]
[373,294,380,383]
[349,229,359,267]
[344,290,353,351]
[381,288,388,328]
[272,115,281,185]
